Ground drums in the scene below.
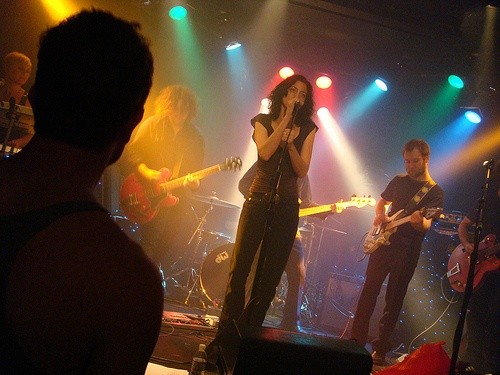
[200,240,259,311]
[195,230,233,265]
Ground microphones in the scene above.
[483,158,497,169]
[9,96,15,113]
[293,100,300,113]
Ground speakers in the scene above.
[389,221,484,366]
[311,271,389,342]
[202,318,373,375]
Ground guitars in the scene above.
[120,156,243,225]
[446,233,500,293]
[295,194,376,238]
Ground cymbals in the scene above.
[191,194,240,210]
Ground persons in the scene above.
[458,183,500,375]
[351,138,445,364]
[1,7,204,375]
[216,74,346,339]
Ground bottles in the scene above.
[189,344,207,375]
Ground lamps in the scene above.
[448,54,473,89]
[155,0,197,22]
[374,59,407,92]
[211,19,243,52]
[457,82,498,125]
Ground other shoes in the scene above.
[281,325,311,334]
[370,350,386,366]
